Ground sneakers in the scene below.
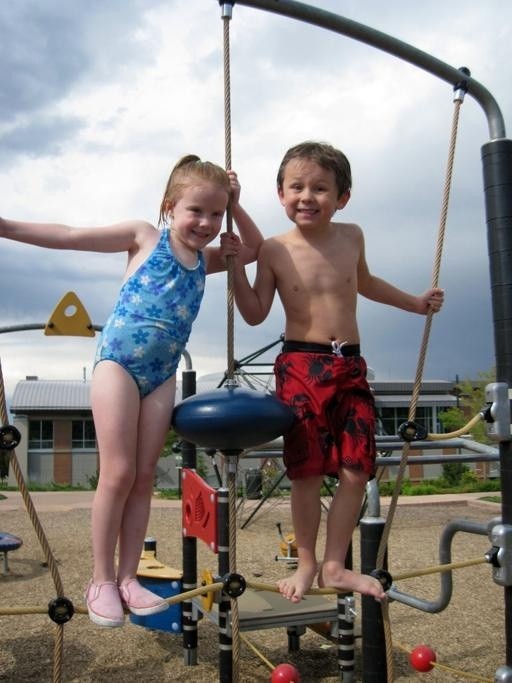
[84,578,129,627]
[118,579,170,616]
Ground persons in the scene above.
[219,142,444,602]
[0,155,264,626]
